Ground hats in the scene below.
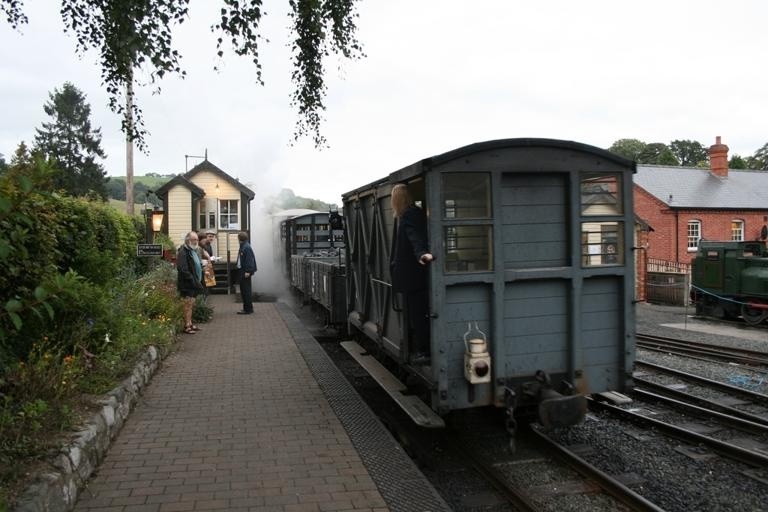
[205,230,216,235]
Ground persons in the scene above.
[390,183,434,366]
[176,230,217,334]
[236,231,257,314]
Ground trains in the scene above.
[688,236,767,326]
[268,138,637,450]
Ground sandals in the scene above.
[183,324,199,334]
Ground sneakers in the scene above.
[412,354,432,365]
[237,309,254,314]
